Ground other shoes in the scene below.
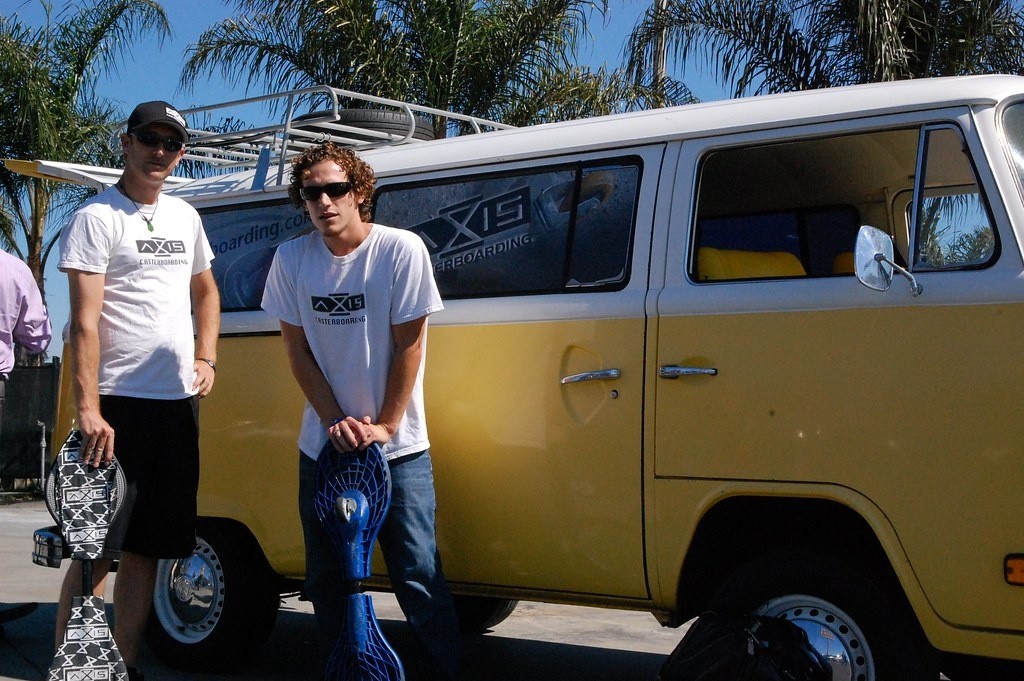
[126,667,144,681]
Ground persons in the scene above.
[54,100,221,681]
[270,140,475,681]
[0,248,52,421]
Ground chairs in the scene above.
[833,252,855,273]
[697,246,806,281]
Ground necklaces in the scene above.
[117,178,159,232]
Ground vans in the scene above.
[0,71,1024,680]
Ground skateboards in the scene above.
[46,429,128,681]
[310,428,406,681]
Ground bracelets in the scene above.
[328,414,347,428]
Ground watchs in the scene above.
[194,358,217,374]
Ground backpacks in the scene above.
[656,611,832,681]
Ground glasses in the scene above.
[129,130,185,152]
[299,182,353,201]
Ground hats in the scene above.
[127,101,189,143]
